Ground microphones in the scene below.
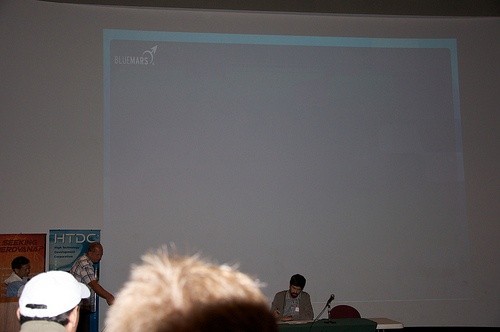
[326,294,334,307]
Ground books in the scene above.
[368,318,404,329]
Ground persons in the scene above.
[102,244,278,332]
[16,270,91,332]
[271,274,314,321]
[6,256,30,297]
[69,242,114,332]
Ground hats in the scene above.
[18,270,91,318]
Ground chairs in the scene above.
[330,304,361,319]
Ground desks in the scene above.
[277,318,378,332]
